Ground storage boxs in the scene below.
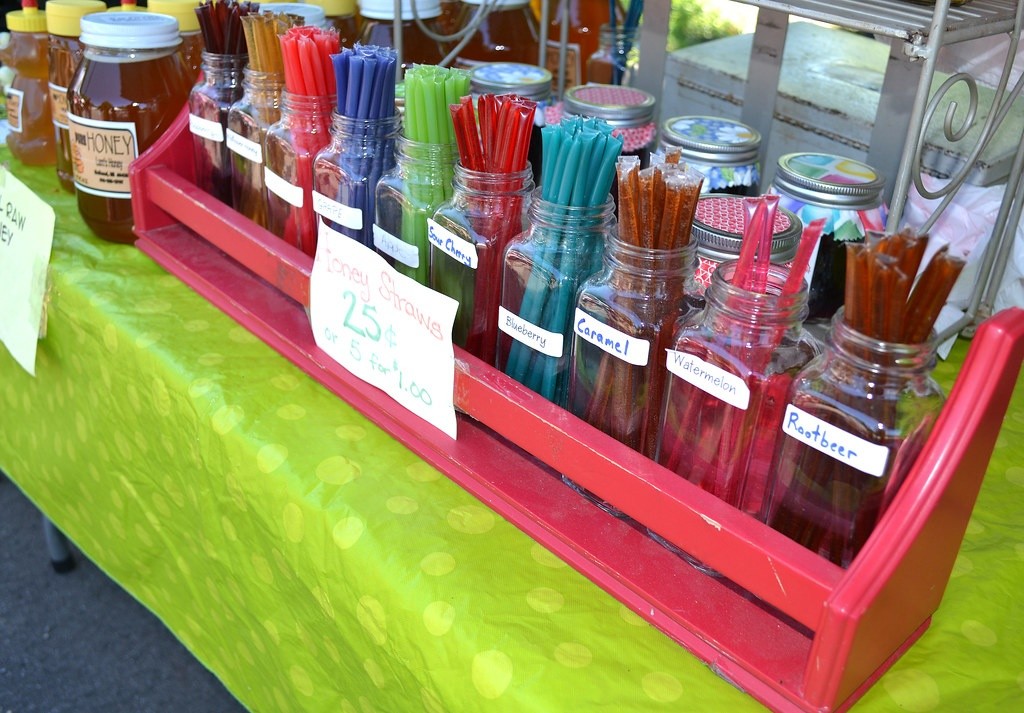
[661,21,1024,195]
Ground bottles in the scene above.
[0,0,948,572]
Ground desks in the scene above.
[0,144,1023,713]
